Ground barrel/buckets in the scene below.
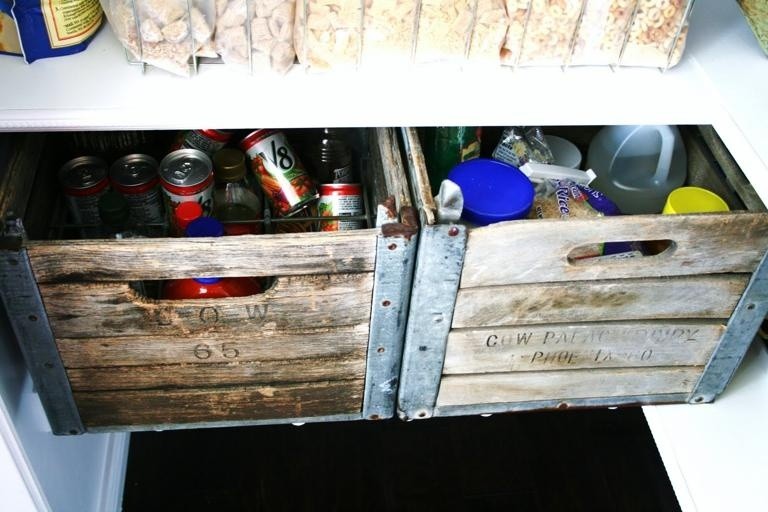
[589,126,687,215]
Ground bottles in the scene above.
[164,219,266,300]
[433,125,483,197]
[319,181,365,233]
[166,128,235,160]
[93,192,159,304]
[170,202,203,239]
[240,128,322,222]
[158,149,217,237]
[109,153,167,236]
[308,126,364,191]
[58,151,111,239]
[212,148,266,235]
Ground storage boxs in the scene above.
[2,129,419,439]
[397,126,764,423]
